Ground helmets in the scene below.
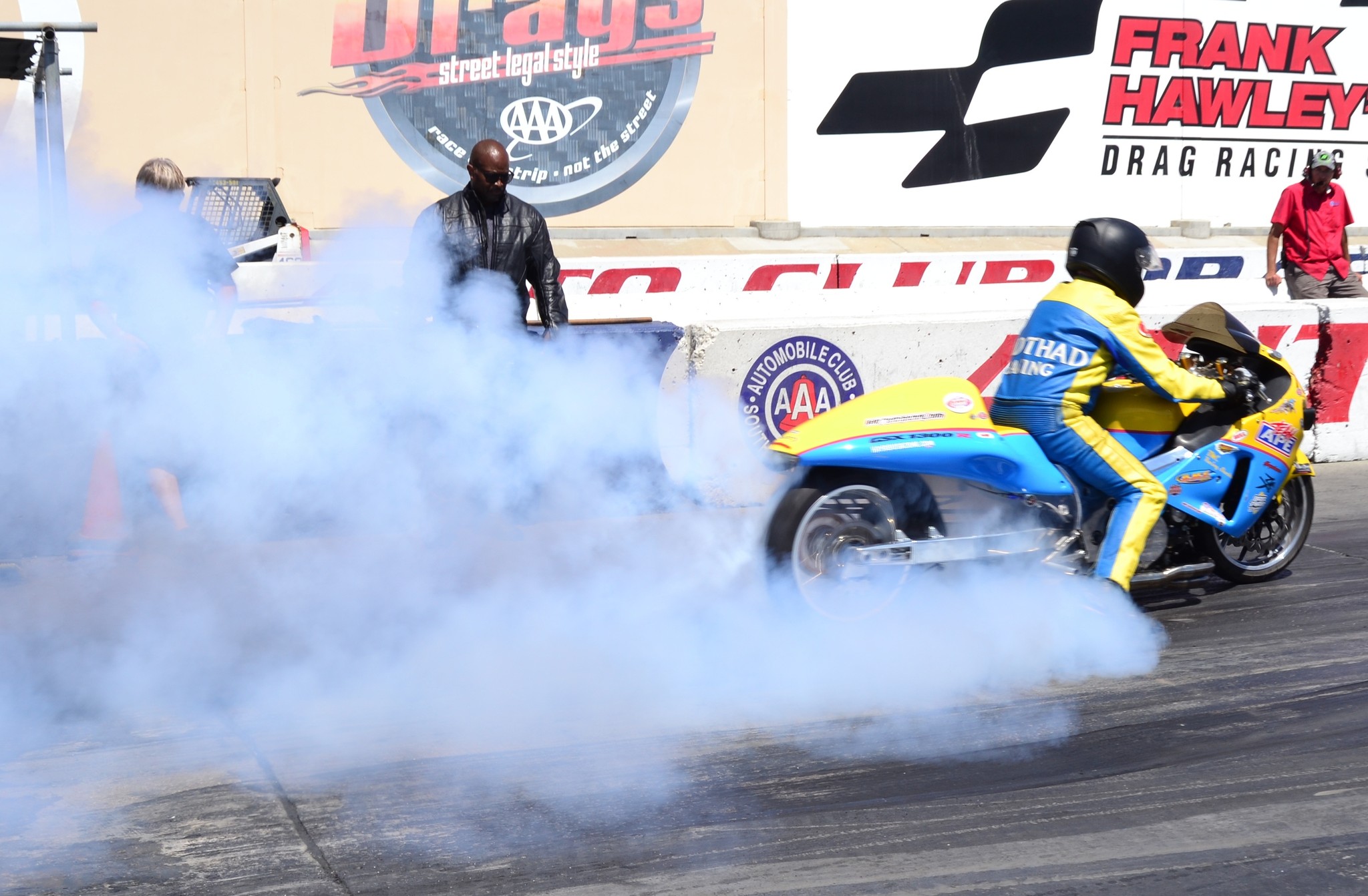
[1066,218,1164,308]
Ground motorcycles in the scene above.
[759,300,1320,653]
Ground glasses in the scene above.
[473,164,514,184]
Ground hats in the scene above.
[1311,151,1336,170]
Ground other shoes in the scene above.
[1081,581,1163,633]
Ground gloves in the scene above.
[1215,366,1260,401]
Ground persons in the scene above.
[991,219,1246,621]
[1263,151,1368,297]
[408,138,569,343]
[84,158,238,329]
